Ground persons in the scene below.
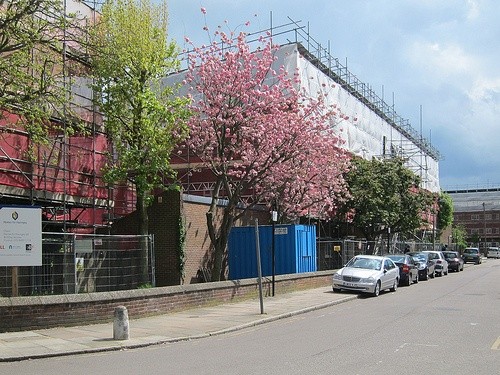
[404,245,410,254]
[442,245,447,251]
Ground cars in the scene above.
[404,253,436,281]
[442,250,465,270]
[461,247,483,263]
[382,254,420,286]
[487,247,500,259]
[421,250,449,276]
[332,256,399,297]
